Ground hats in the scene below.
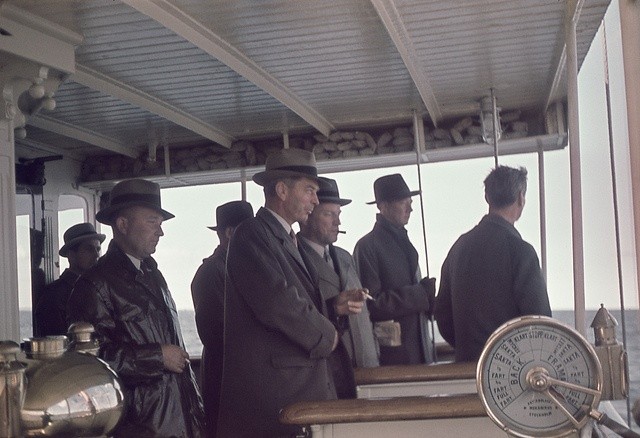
[252,148,332,192]
[58,223,105,257]
[316,177,351,206]
[97,179,175,225]
[208,202,253,230]
[366,174,420,204]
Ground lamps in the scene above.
[480,95,501,144]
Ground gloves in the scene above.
[420,277,436,300]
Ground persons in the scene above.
[216,148,370,437]
[65,179,209,437]
[437,166,552,362]
[294,177,375,368]
[353,174,438,367]
[30,227,47,303]
[191,201,253,437]
[33,222,106,338]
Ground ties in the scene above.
[323,247,334,267]
[140,262,156,291]
[290,230,298,248]
